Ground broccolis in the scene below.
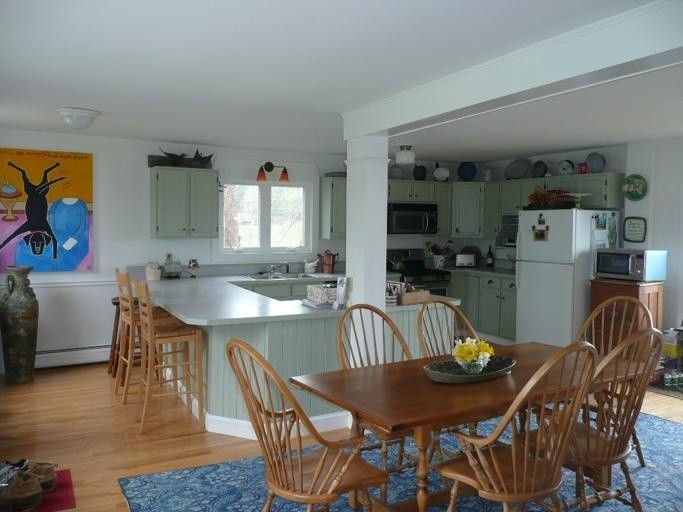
[515,208,621,349]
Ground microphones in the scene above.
[107,295,159,379]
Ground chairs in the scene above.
[417,299,517,461]
[113,267,180,404]
[518,329,665,512]
[131,277,206,434]
[225,337,389,511]
[436,342,599,511]
[562,296,653,468]
[336,304,448,504]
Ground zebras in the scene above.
[411,281,450,297]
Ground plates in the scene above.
[450,269,518,342]
[548,173,625,210]
[387,179,436,204]
[499,177,548,217]
[422,181,453,236]
[480,180,512,238]
[588,278,665,366]
[318,175,346,243]
[454,181,484,240]
[148,166,222,241]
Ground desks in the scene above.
[290,341,664,511]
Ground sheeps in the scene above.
[270,261,290,275]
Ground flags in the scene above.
[0,263,40,385]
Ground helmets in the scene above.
[586,152,606,173]
[557,159,573,177]
[530,160,548,178]
[413,165,427,181]
[457,162,478,180]
[389,164,408,180]
[433,167,450,182]
[504,158,529,180]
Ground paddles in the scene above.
[117,400,683,512]
[30,469,76,511]
[646,383,682,400]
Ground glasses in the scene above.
[395,143,415,166]
[255,162,290,184]
[56,107,102,130]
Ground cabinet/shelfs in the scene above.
[454,181,484,240]
[450,269,518,342]
[548,173,625,210]
[485,245,494,269]
[318,175,346,243]
[0,265,38,384]
[422,181,453,236]
[588,278,665,366]
[499,177,548,217]
[148,166,222,241]
[387,179,436,204]
[480,180,512,238]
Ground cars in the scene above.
[386,295,398,305]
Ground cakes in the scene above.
[578,163,587,173]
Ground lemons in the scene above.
[389,260,407,272]
[483,167,497,181]
[316,249,339,275]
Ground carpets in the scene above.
[117,400,683,512]
[646,383,682,400]
[30,469,76,511]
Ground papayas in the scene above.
[451,254,475,267]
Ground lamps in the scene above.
[113,267,180,404]
[56,107,102,130]
[255,162,290,184]
[395,143,415,166]
[131,277,206,434]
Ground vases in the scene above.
[0,263,40,385]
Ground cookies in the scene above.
[388,203,437,235]
[593,248,667,283]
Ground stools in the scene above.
[107,295,159,379]
